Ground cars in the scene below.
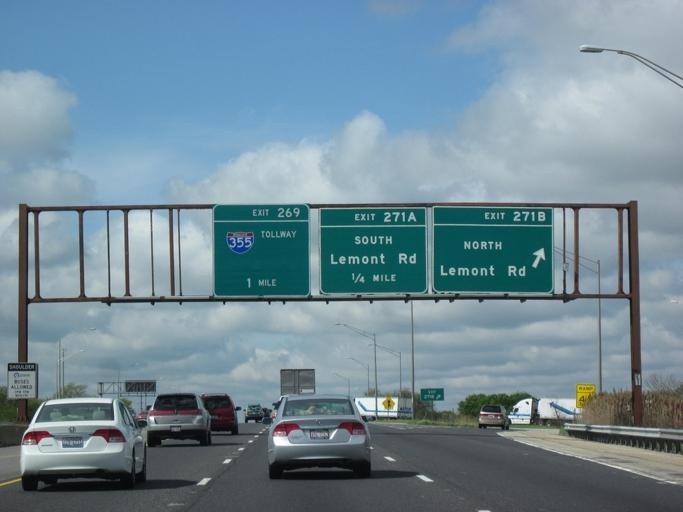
[478,404,509,430]
[262,393,371,479]
[243,403,272,423]
[19,392,242,490]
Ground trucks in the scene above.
[280,368,316,395]
[508,398,583,427]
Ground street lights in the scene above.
[368,343,402,392]
[335,322,378,421]
[579,45,683,88]
[57,327,97,399]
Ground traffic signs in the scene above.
[420,388,444,400]
[431,206,553,293]
